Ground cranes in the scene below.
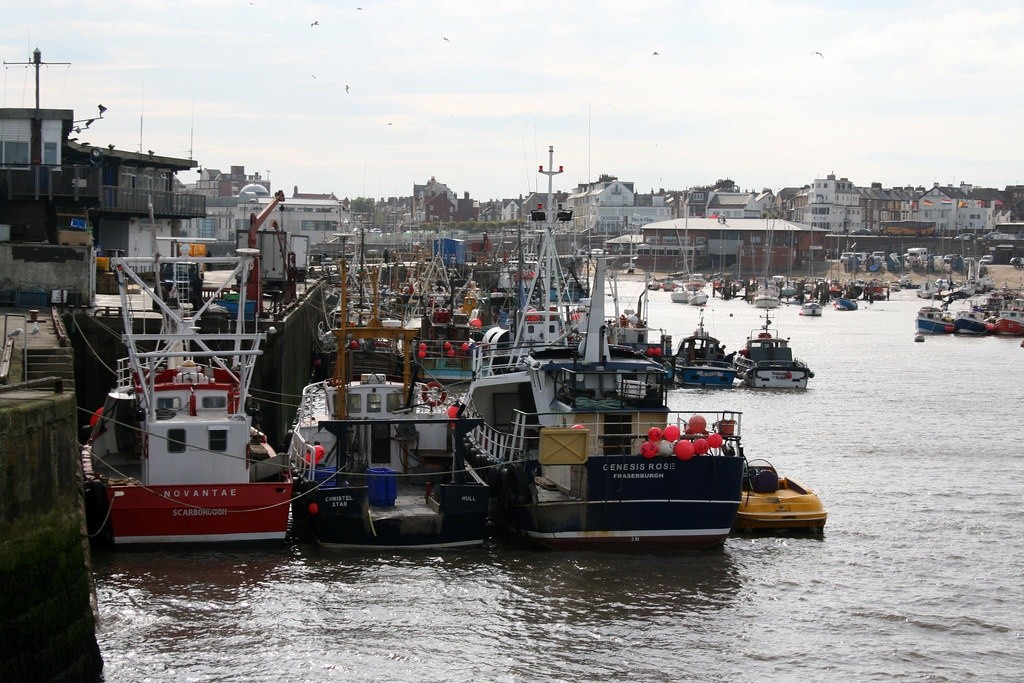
[244,188,286,319]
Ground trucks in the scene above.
[907,248,928,263]
[291,234,311,283]
[235,229,297,295]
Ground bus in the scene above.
[877,220,936,236]
[994,223,1024,241]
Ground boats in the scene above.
[80,142,1024,553]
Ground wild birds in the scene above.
[651,50,661,57]
[310,20,319,28]
[344,85,351,94]
[443,37,450,42]
[387,122,393,126]
[311,74,316,80]
[810,51,824,60]
[356,7,363,11]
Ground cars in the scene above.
[965,256,974,267]
[980,254,994,265]
[953,233,974,242]
[851,228,871,235]
[944,255,953,262]
[982,232,999,240]
[851,252,864,264]
[872,251,884,262]
[840,253,853,264]
